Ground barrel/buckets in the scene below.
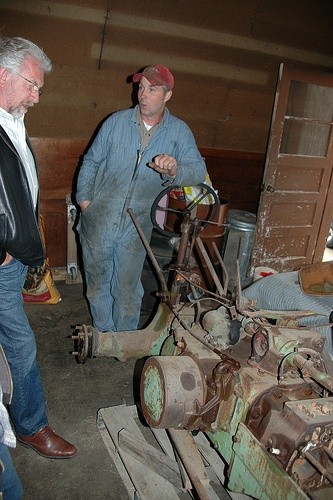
[221,208,257,291]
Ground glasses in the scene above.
[0,66,44,96]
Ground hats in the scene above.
[133,64,175,89]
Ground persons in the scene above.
[75,64,207,335]
[0,343,28,500]
[0,34,78,460]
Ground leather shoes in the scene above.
[16,426,77,459]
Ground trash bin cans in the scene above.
[222,209,257,290]
[254,266,279,282]
